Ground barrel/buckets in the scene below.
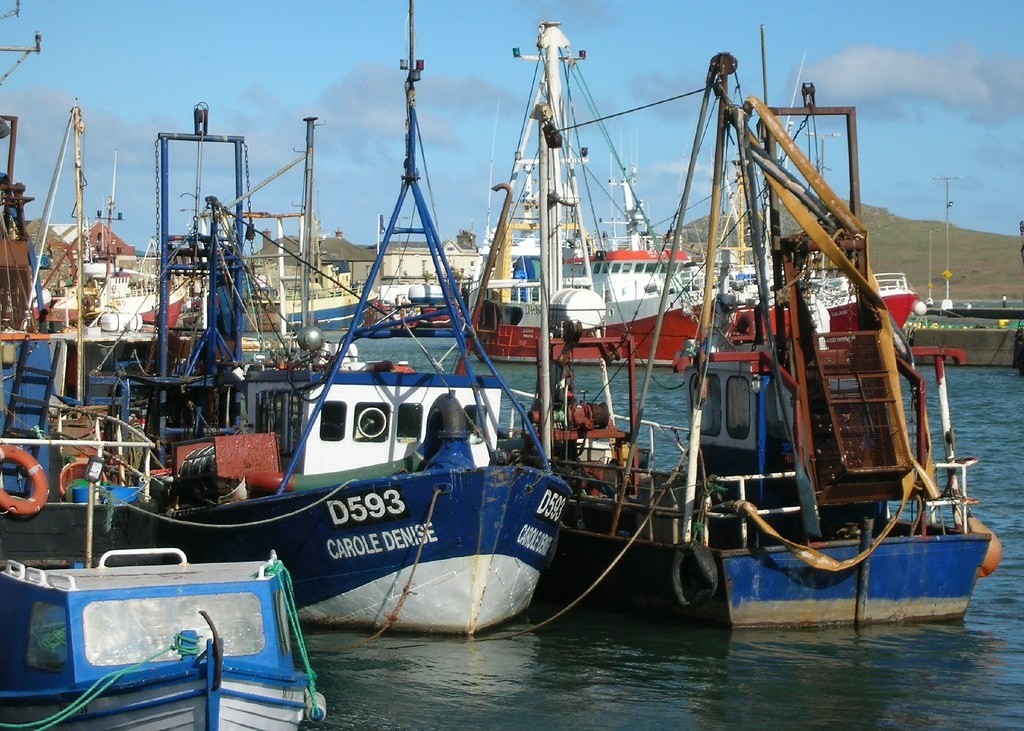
[72,486,139,503]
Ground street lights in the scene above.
[932,176,964,310]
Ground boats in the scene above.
[0,0,1004,730]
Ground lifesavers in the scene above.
[0,443,50,519]
[672,541,719,610]
